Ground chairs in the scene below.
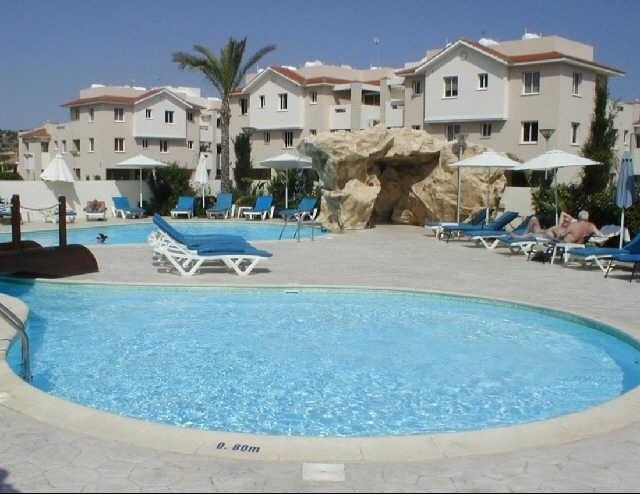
[423,206,639,285]
[53,203,76,224]
[86,200,105,222]
[171,192,318,221]
[1,199,14,222]
[147,210,275,277]
[113,196,145,219]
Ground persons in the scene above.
[510,211,577,239]
[561,210,603,244]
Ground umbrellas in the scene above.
[116,155,168,208]
[40,155,74,183]
[448,152,521,225]
[505,148,605,226]
[194,156,209,208]
[260,154,313,209]
[615,150,637,249]
